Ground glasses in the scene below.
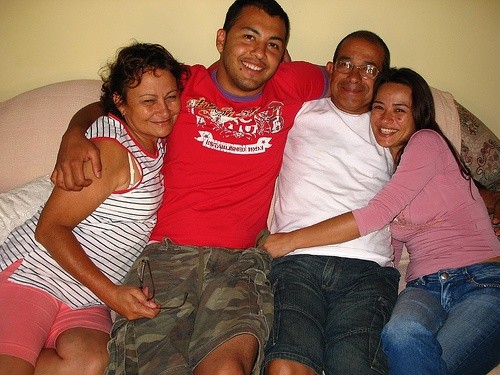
[136,256,189,309]
[332,55,384,81]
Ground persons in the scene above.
[49,0,332,375]
[0,44,293,375]
[255,68,500,375]
[259,31,500,375]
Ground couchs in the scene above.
[0,79,500,244]
